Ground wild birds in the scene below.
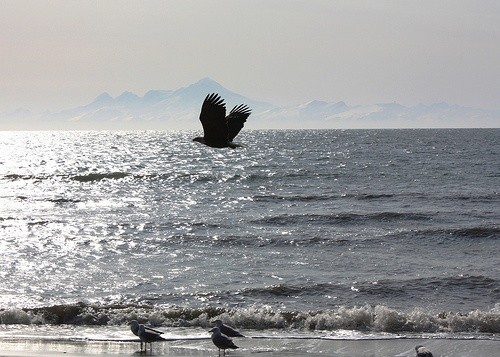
[208,327,240,357]
[211,320,245,340]
[127,320,164,353]
[137,324,166,353]
[192,92,253,150]
[415,344,433,357]
